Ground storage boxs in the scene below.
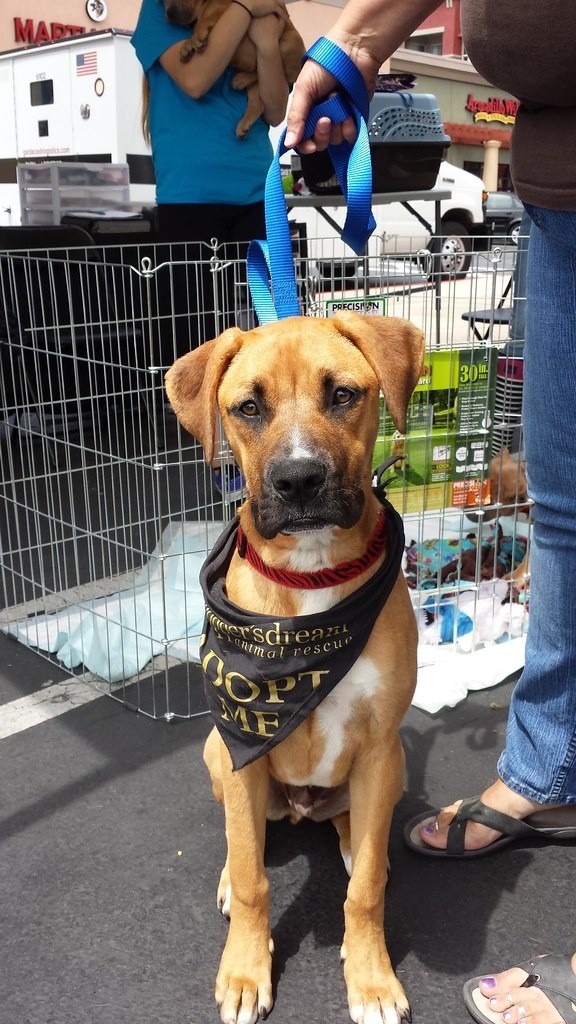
[295,92,452,197]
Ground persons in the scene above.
[286,0,576,1024]
[132,0,307,504]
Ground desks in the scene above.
[286,188,450,348]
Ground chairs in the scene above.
[462,274,514,342]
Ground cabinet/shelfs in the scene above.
[16,161,131,228]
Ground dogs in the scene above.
[163,310,428,1024]
[462,447,533,605]
[165,0,306,139]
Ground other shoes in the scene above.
[212,465,247,501]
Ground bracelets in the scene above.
[230,0,255,20]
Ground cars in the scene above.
[482,192,527,248]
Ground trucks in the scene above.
[0,31,488,296]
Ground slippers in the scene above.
[404,794,576,859]
[462,951,576,1024]
